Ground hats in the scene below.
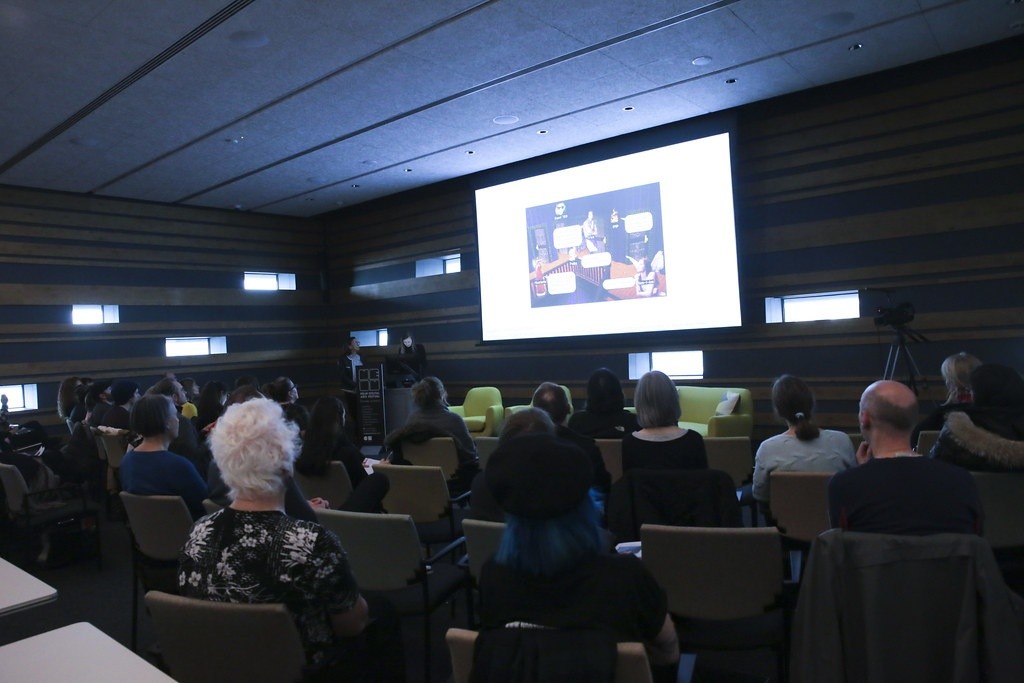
[92,379,113,394]
[487,435,594,519]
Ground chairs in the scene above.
[0,433,1024,682]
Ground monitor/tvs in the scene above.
[385,351,419,375]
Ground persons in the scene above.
[582,211,601,253]
[6,331,1023,669]
[635,259,657,298]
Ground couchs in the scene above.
[447,387,502,437]
[624,386,755,487]
[506,386,573,433]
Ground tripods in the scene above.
[881,327,947,412]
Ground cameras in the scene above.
[874,301,914,327]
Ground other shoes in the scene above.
[47,436,62,445]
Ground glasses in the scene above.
[288,384,298,391]
[352,342,360,345]
